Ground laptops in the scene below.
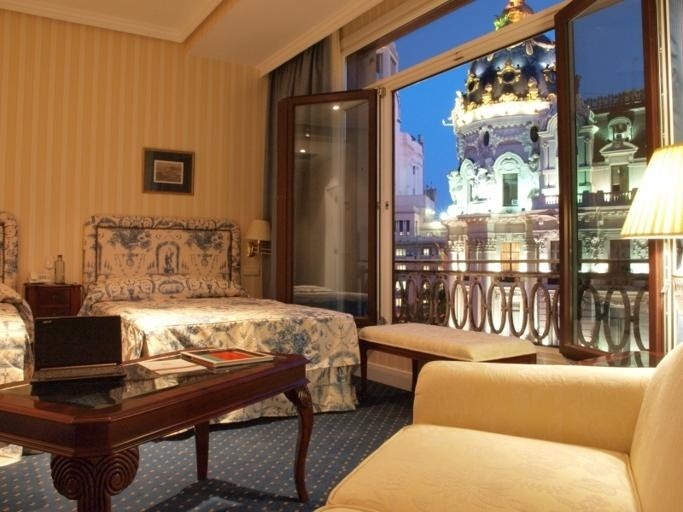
[29,315,127,387]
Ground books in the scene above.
[138,359,208,379]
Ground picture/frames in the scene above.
[142,145,196,198]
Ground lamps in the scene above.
[618,143,682,243]
[245,216,272,259]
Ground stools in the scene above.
[360,321,535,407]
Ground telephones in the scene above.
[27,271,49,284]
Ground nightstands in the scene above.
[23,279,82,315]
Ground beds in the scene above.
[294,283,367,309]
[0,212,33,381]
[81,216,358,412]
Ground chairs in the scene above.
[326,339,683,508]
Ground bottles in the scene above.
[55,254,66,285]
